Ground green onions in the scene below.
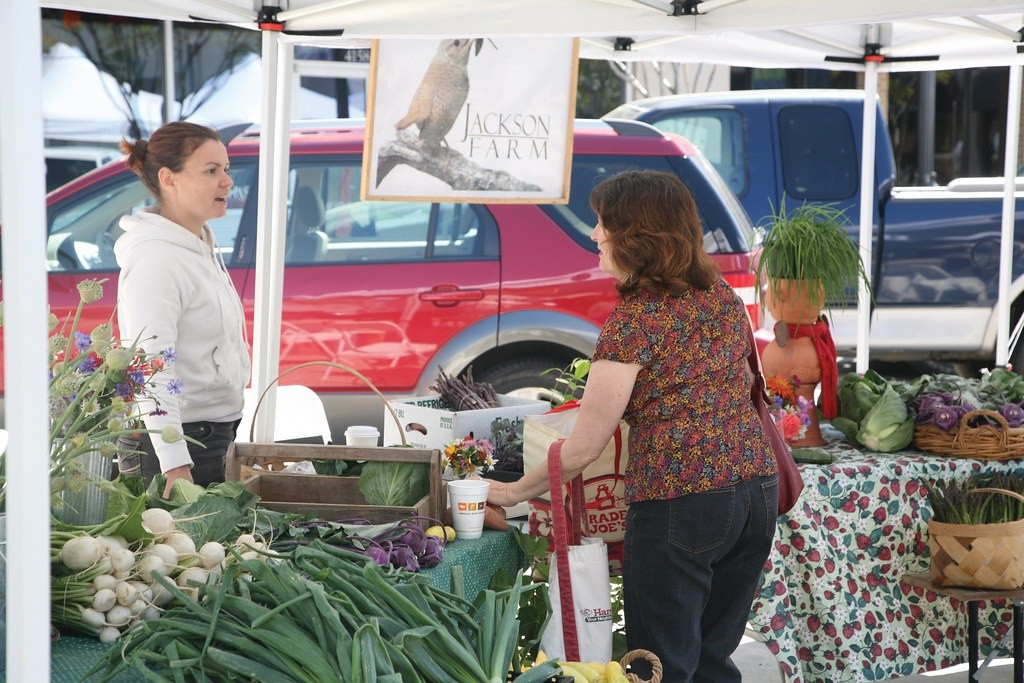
[80,538,578,683]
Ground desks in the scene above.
[748,425,1024,683]
[0,529,529,683]
[901,574,1024,683]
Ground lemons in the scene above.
[424,525,456,543]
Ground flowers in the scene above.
[48,331,182,438]
[760,373,814,440]
[443,435,499,480]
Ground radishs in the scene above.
[55,509,284,644]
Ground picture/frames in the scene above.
[361,38,580,205]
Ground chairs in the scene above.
[292,186,329,262]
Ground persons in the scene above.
[113,122,251,499]
[467,169,778,683]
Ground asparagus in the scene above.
[427,362,501,412]
[918,466,1024,524]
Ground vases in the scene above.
[49,439,117,526]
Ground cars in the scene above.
[44,146,125,196]
[0,118,772,485]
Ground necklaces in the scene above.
[902,71,964,182]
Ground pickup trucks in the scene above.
[320,90,1023,380]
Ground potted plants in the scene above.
[745,189,880,331]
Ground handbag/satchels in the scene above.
[535,441,612,663]
[736,295,804,515]
[522,403,634,542]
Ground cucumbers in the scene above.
[790,450,832,465]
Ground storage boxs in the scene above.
[383,393,551,458]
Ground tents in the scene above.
[1,0,1024,683]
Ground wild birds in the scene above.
[393,38,484,173]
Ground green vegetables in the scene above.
[311,440,447,507]
[834,369,1024,452]
[104,472,312,550]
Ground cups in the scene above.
[447,479,490,540]
[344,425,380,463]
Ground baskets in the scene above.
[242,362,406,483]
[914,409,1024,462]
[927,488,1024,590]
